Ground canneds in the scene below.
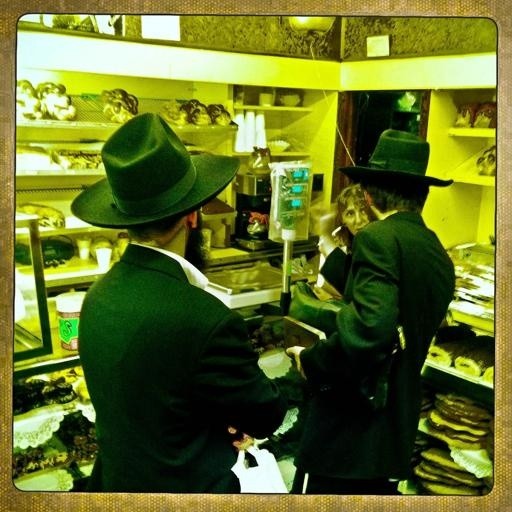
[55,291,88,351]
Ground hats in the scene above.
[339,129,453,186]
[70,112,242,228]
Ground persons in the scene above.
[312,183,380,300]
[69,110,288,494]
[284,128,456,494]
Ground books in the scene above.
[284,314,328,364]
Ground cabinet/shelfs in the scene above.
[14,25,495,494]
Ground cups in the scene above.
[234,109,269,154]
[77,237,91,260]
[96,248,112,270]
[281,95,300,106]
[259,93,273,107]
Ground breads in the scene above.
[427,345,494,386]
[14,75,77,123]
[411,393,494,496]
[13,362,101,481]
[158,97,235,127]
[16,201,64,229]
[101,86,138,127]
[15,146,103,168]
